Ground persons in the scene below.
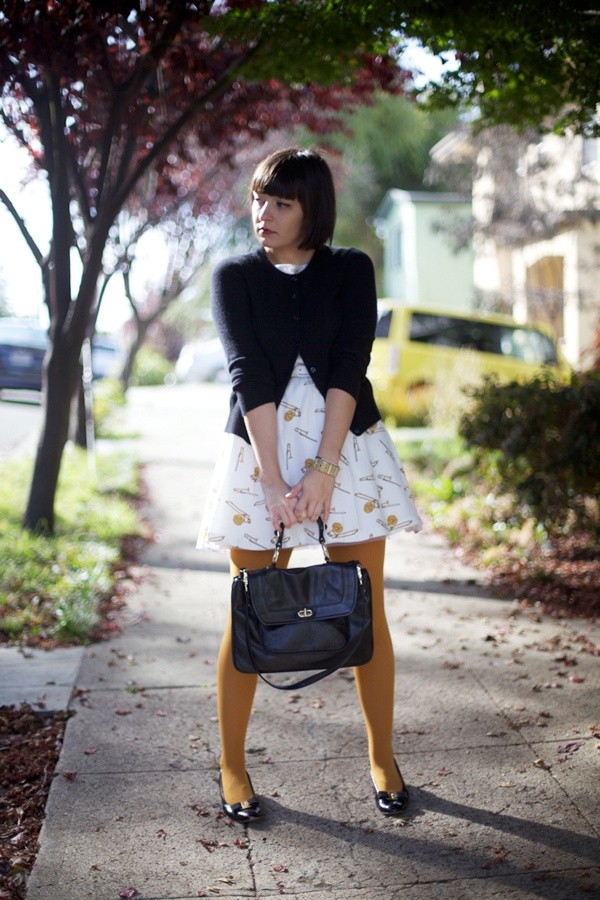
[196,145,423,828]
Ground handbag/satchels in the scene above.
[230,513,374,691]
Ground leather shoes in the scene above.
[370,757,410,816]
[218,768,263,824]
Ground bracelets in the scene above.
[311,456,341,478]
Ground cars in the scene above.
[364,298,574,430]
[0,315,123,393]
